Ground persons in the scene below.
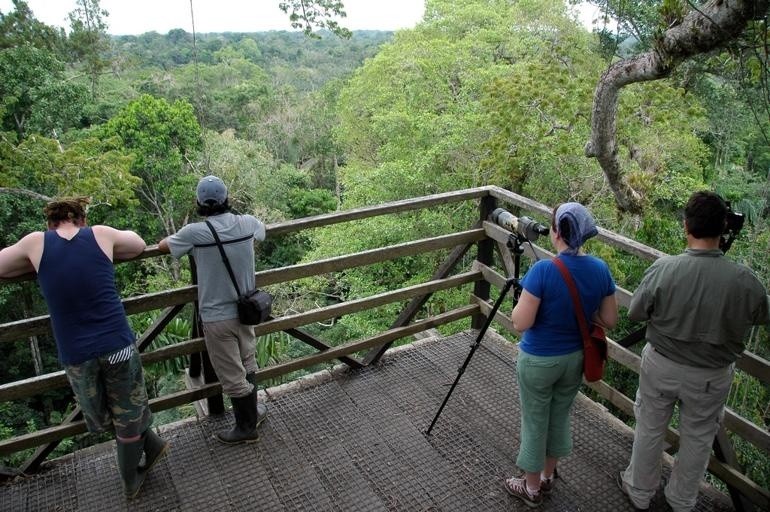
[0,195,169,498]
[620,191,770,511]
[159,175,268,445]
[504,203,617,508]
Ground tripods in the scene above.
[427,249,523,437]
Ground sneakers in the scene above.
[539,472,554,495]
[504,476,544,508]
[617,471,649,510]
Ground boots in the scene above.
[215,388,260,446]
[137,428,171,473]
[114,436,147,500]
[247,371,267,428]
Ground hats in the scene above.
[196,175,228,206]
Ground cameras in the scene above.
[722,201,744,234]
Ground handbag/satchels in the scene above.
[580,324,609,382]
[237,291,272,324]
